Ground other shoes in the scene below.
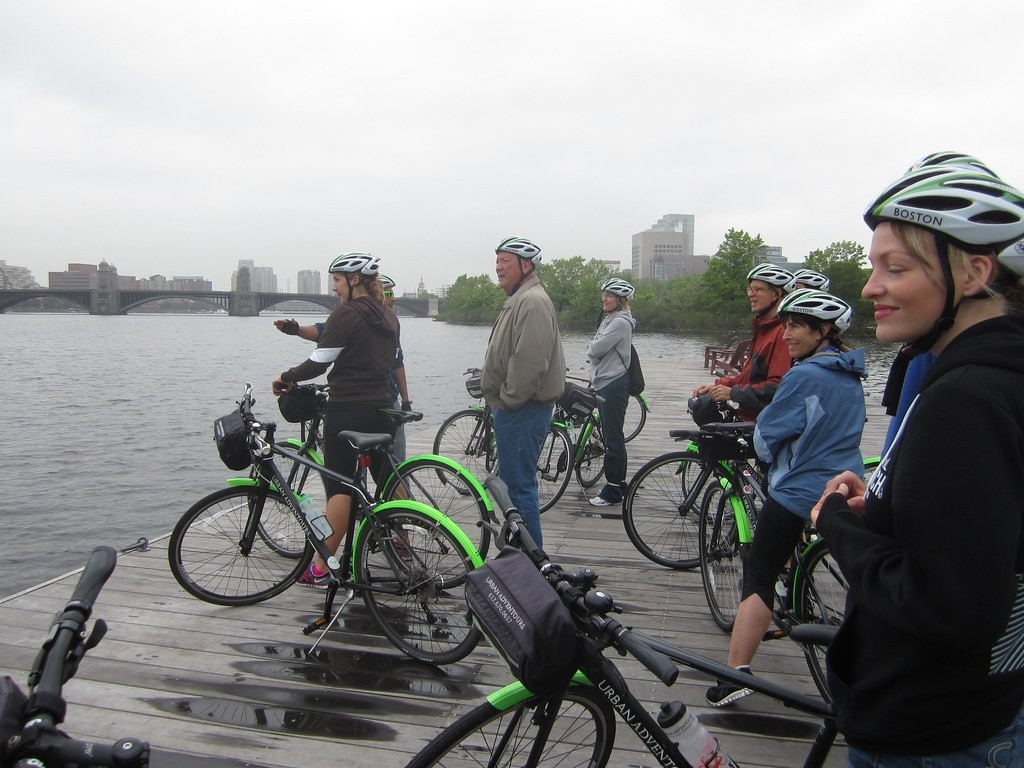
[394,543,412,560]
[589,497,623,506]
[295,562,332,589]
[596,490,624,499]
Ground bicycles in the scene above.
[245,382,501,590]
[622,369,883,571]
[166,382,486,668]
[430,366,501,497]
[0,544,150,768]
[665,421,852,703]
[401,479,846,768]
[534,360,650,515]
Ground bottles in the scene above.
[565,421,577,444]
[657,701,741,768]
[298,494,333,541]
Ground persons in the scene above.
[272,251,413,589]
[586,278,637,507]
[691,263,830,605]
[811,150,1024,768]
[480,235,566,550]
[706,289,868,705]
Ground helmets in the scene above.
[863,165,1024,254]
[329,253,381,275]
[903,151,1000,179]
[777,288,851,331]
[495,236,542,266]
[601,278,635,300]
[375,274,396,288]
[747,263,796,292]
[793,269,829,292]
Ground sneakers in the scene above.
[706,665,754,706]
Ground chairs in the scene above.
[704,335,752,377]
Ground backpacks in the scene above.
[617,318,645,396]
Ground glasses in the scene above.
[747,286,764,294]
